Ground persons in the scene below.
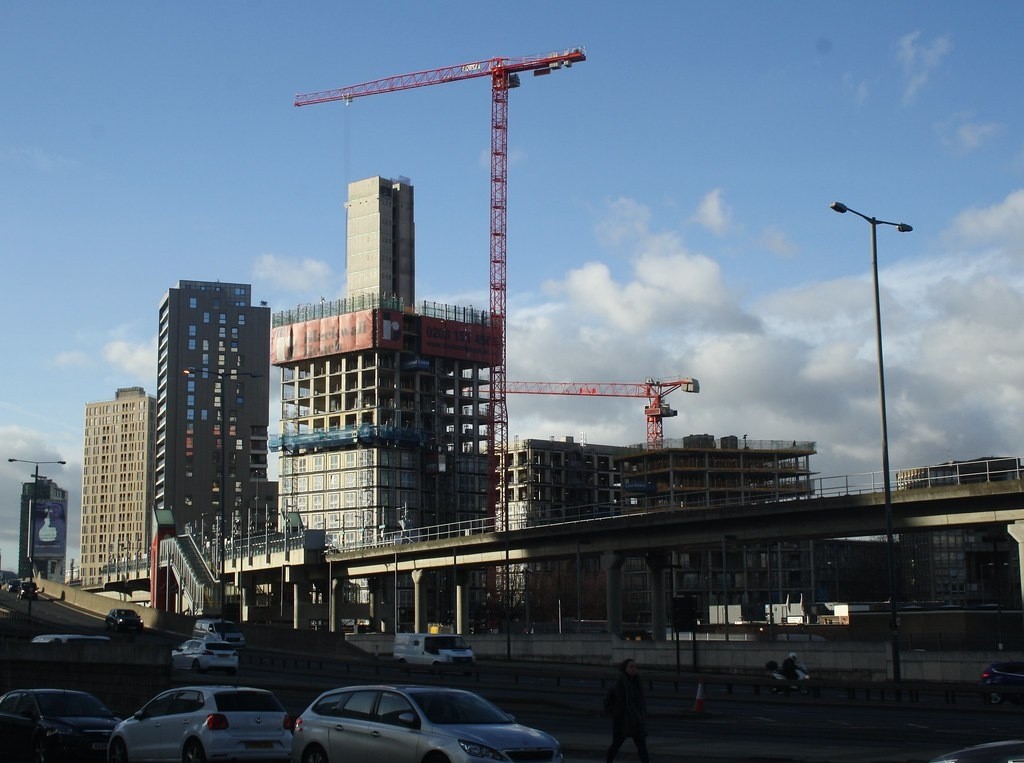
[782,653,798,679]
[606,658,652,763]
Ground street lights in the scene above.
[184,369,265,644]
[9,456,66,582]
[831,201,914,701]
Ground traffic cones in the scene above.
[690,676,706,713]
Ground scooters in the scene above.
[764,661,811,694]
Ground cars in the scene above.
[29,633,111,644]
[17,582,39,600]
[0,687,123,762]
[979,661,1024,706]
[5,579,20,591]
[107,683,293,763]
[104,609,144,632]
[170,639,239,673]
[290,683,565,763]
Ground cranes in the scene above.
[290,46,589,531]
[451,376,699,453]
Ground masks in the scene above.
[626,668,637,675]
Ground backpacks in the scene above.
[603,687,616,714]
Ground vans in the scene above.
[393,632,477,674]
[191,617,244,650]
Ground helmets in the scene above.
[789,652,797,662]
